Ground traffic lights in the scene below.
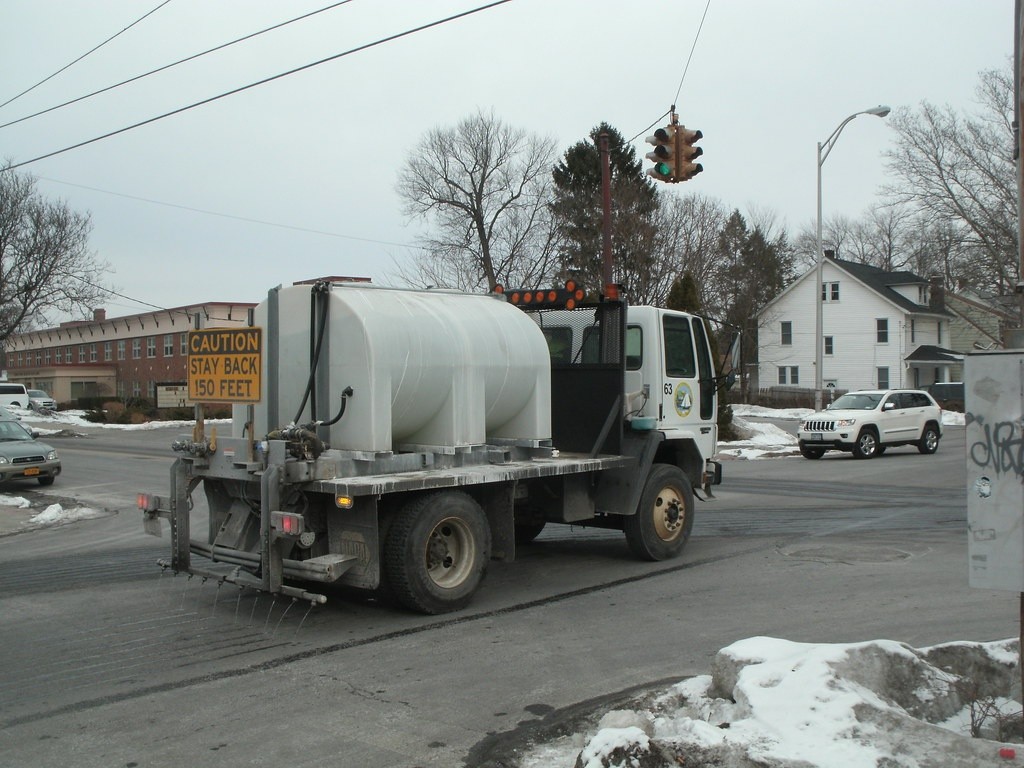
[675,123,704,183]
[651,125,678,183]
[644,133,658,179]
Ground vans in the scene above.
[0,383,30,411]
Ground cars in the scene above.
[0,405,62,487]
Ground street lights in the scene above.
[817,103,892,411]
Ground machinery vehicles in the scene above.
[136,280,744,615]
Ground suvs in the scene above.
[27,389,59,413]
[796,388,944,459]
